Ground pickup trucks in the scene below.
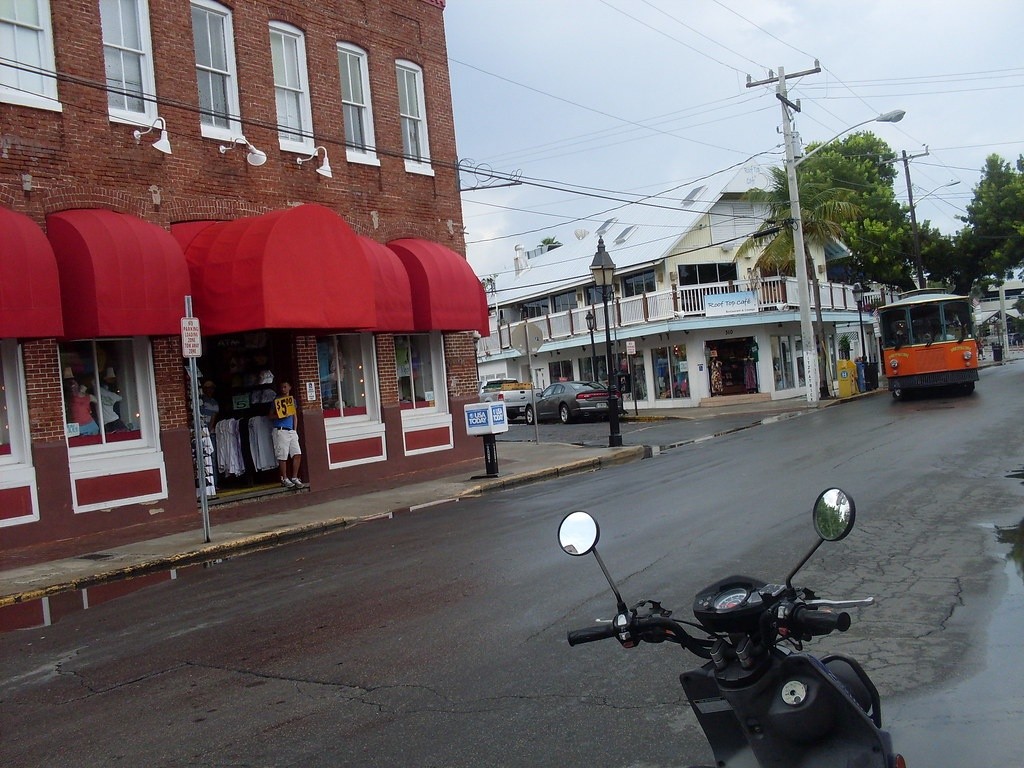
[477,378,542,419]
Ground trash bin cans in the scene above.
[856,362,879,392]
[992,345,1002,361]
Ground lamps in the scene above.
[133,117,173,155]
[296,146,332,179]
[220,137,267,166]
[721,242,736,252]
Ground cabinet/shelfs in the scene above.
[229,345,276,417]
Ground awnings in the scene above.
[668,311,874,337]
[0,203,489,339]
[477,312,671,367]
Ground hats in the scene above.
[204,381,216,388]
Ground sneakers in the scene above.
[290,478,305,489]
[281,475,295,488]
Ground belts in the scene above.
[274,427,291,430]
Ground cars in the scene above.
[1008,333,1022,345]
[525,381,623,424]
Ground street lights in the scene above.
[473,330,481,381]
[908,179,961,288]
[784,110,907,403]
[852,283,872,392]
[585,311,599,382]
[589,236,623,447]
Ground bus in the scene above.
[877,287,979,400]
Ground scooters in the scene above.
[557,487,908,768]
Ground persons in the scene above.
[70,379,100,436]
[395,337,410,377]
[326,353,348,410]
[92,380,129,431]
[269,382,305,488]
[977,338,985,360]
